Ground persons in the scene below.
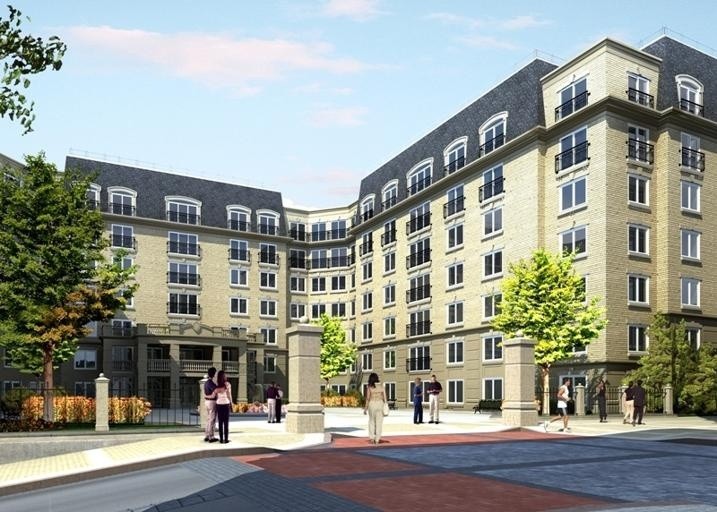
[202,367,226,441]
[413,377,423,424]
[265,380,279,423]
[622,381,634,423]
[274,385,283,422]
[631,379,645,426]
[205,370,233,443]
[543,377,571,432]
[597,380,607,422]
[362,372,386,445]
[425,374,441,424]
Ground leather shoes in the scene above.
[370,439,380,445]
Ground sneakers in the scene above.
[204,437,230,443]
[563,427,572,434]
[543,419,551,430]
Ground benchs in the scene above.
[471,398,502,415]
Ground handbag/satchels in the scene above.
[382,402,390,417]
